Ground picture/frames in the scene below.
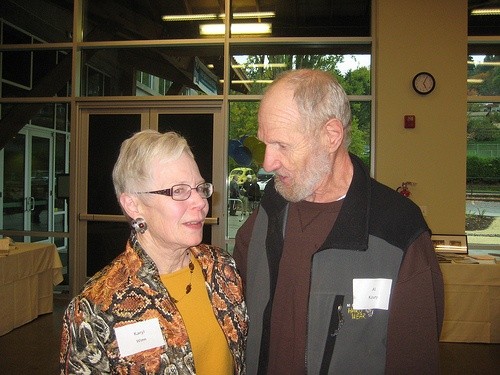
[431,234,468,255]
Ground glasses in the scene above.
[134,183,214,202]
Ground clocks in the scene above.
[412,72,435,95]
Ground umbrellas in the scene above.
[228,135,266,167]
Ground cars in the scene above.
[230,167,256,185]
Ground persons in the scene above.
[239,175,261,222]
[229,175,239,216]
[234,69,444,375]
[59,129,249,375]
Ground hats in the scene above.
[247,175,251,179]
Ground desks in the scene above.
[435,253,500,343]
[0,242,63,336]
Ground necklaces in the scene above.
[169,250,194,303]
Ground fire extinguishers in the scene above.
[395,181,416,197]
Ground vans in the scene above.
[257,168,275,195]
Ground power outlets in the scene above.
[420,206,427,217]
